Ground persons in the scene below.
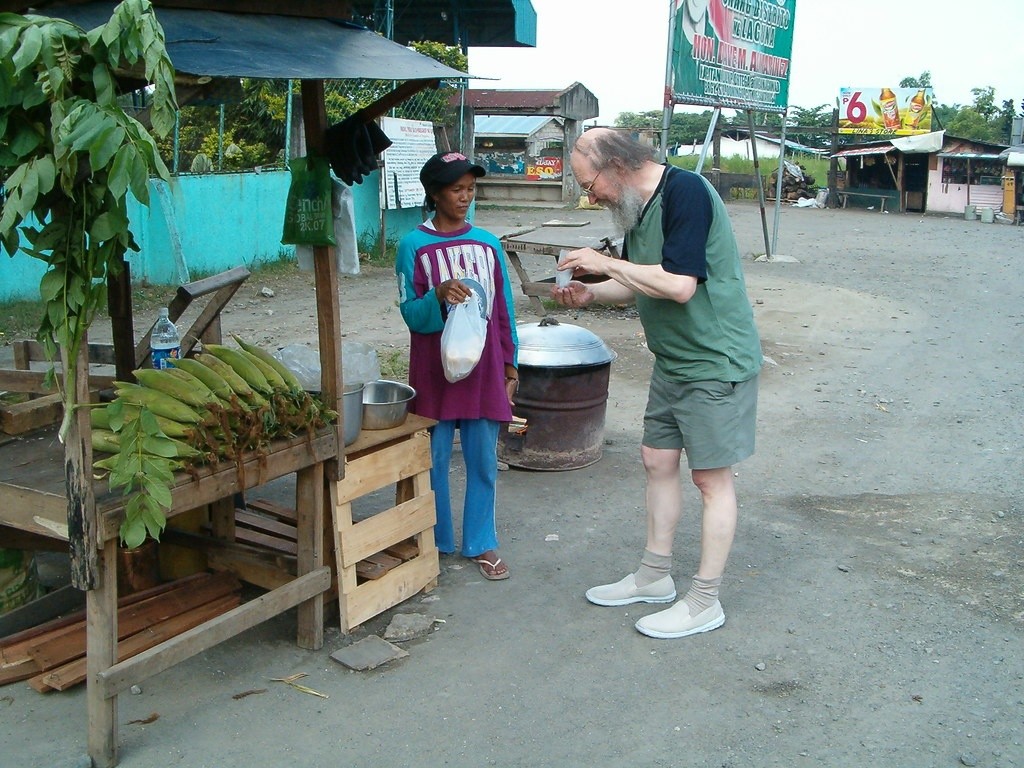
[395,152,519,579]
[550,128,760,638]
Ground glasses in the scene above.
[583,168,602,195]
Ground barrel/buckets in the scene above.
[964,205,977,220]
[981,207,994,223]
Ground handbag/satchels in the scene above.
[280,156,338,248]
[440,288,488,383]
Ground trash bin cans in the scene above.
[964,205,978,220]
[981,207,994,223]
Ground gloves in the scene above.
[331,108,392,186]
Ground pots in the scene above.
[306,382,366,446]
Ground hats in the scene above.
[420,152,486,194]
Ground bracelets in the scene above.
[507,377,519,391]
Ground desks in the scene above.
[224,375,441,634]
[499,219,620,318]
[0,339,333,768]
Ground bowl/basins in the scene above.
[361,379,418,430]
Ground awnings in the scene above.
[36,0,473,79]
[832,146,897,186]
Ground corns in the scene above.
[85,331,340,471]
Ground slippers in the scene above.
[470,552,510,579]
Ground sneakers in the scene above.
[634,600,727,639]
[585,572,677,606]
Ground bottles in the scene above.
[903,88,925,130]
[149,307,181,369]
[879,88,901,129]
